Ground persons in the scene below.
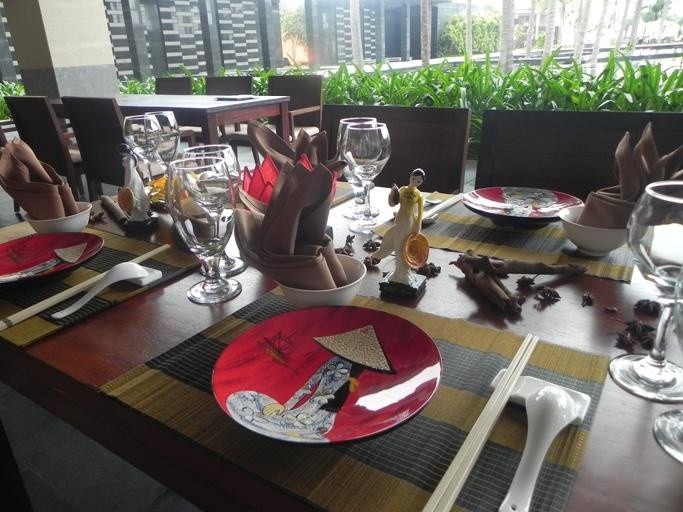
[116,143,158,233]
[364,168,430,302]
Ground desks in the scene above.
[51,95,291,157]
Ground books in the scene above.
[217,94,253,100]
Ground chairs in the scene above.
[206,76,252,167]
[321,105,470,194]
[60,95,199,203]
[227,75,322,174]
[475,107,683,226]
[2,95,84,213]
[156,77,192,95]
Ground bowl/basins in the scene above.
[25,202,92,233]
[275,255,366,305]
[559,206,629,254]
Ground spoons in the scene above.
[498,387,578,512]
[52,262,148,319]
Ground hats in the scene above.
[402,233,431,270]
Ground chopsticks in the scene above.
[0,244,170,332]
[423,194,464,217]
[421,334,540,512]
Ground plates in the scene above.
[211,305,442,445]
[462,187,583,228]
[1,232,105,284]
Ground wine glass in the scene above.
[182,144,246,278]
[337,117,383,218]
[145,111,180,176]
[609,181,683,402]
[123,114,162,183]
[342,122,391,234]
[168,158,243,303]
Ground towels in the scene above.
[578,122,680,227]
[248,119,329,163]
[2,137,79,220]
[234,162,351,291]
